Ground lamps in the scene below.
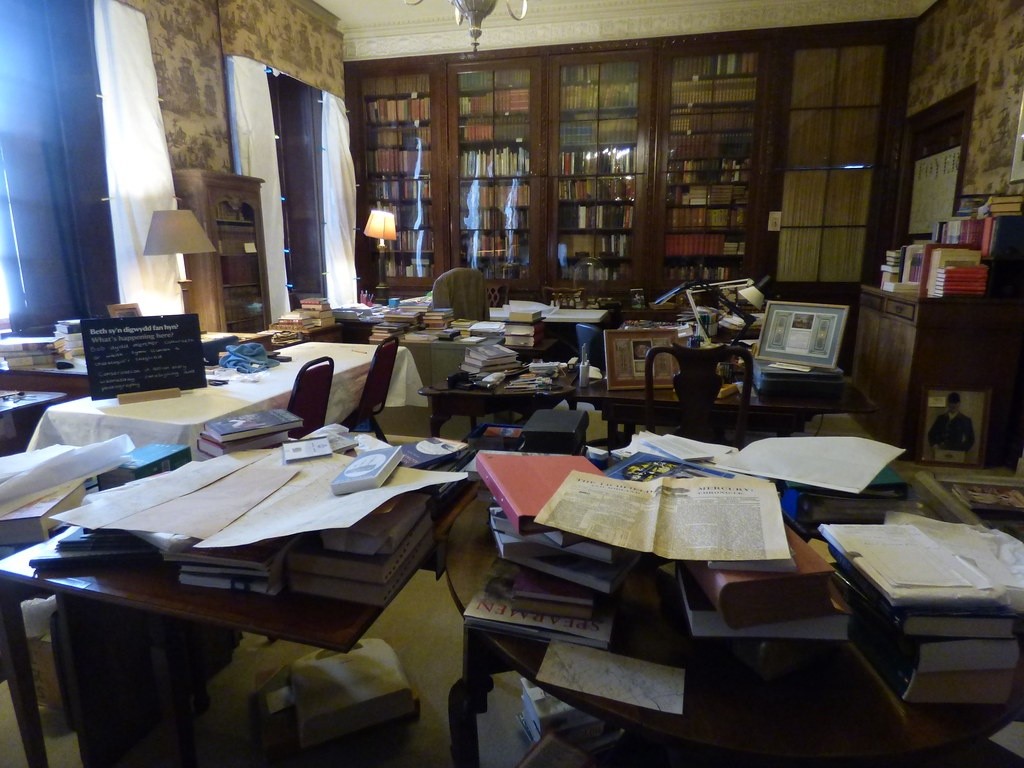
[407,0,528,54]
[364,210,396,306]
[686,278,763,351]
[143,210,218,315]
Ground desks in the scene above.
[0,296,1024,768]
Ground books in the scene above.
[269,297,336,333]
[365,296,609,393]
[512,675,625,768]
[358,74,437,279]
[466,409,907,657]
[458,70,533,280]
[0,408,475,610]
[818,511,1024,710]
[878,239,989,297]
[665,52,757,283]
[932,190,1024,256]
[3,318,88,369]
[556,63,639,283]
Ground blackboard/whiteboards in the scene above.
[82,313,208,400]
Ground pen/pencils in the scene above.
[506,378,560,391]
[362,289,374,301]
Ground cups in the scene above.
[389,298,400,308]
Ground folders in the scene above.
[476,452,607,535]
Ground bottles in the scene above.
[551,293,575,309]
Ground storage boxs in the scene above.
[520,409,588,454]
[461,421,525,450]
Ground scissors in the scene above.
[209,377,240,386]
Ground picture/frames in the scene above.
[917,387,996,468]
[754,301,849,369]
[602,326,677,391]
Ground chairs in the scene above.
[640,342,755,451]
[434,268,492,323]
[574,322,604,370]
[285,356,334,439]
[338,337,398,442]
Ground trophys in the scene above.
[412,0,523,52]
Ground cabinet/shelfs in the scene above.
[172,169,272,334]
[851,286,1024,460]
[343,29,786,302]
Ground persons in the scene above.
[928,392,975,452]
[636,344,648,357]
